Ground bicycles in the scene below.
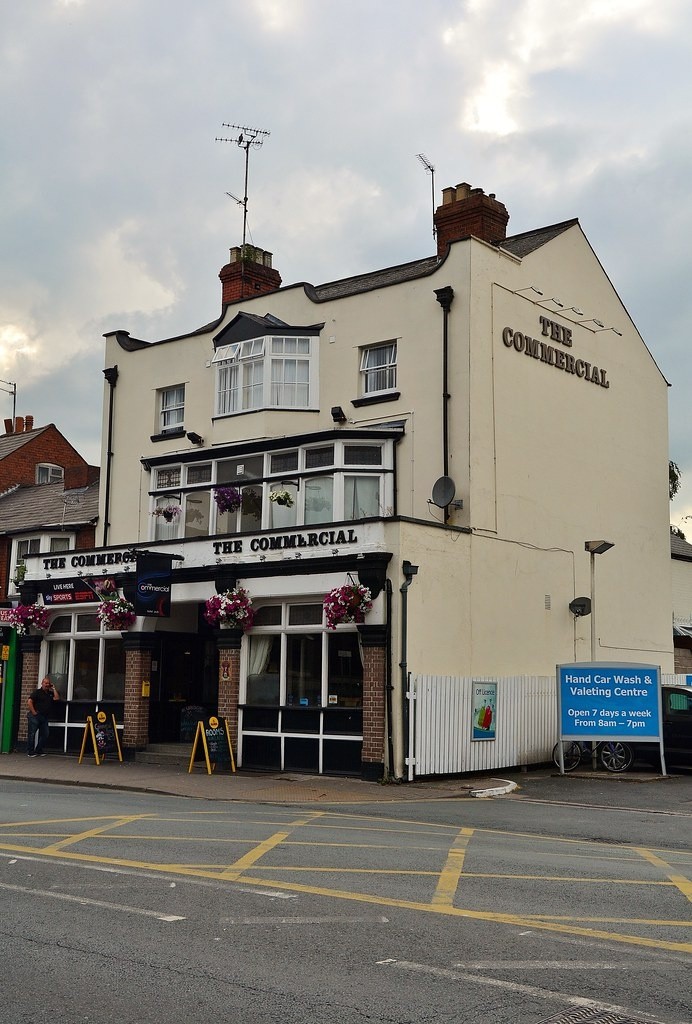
[551,739,635,773]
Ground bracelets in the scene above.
[52,688,56,691]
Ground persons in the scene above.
[27,677,59,757]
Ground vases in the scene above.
[341,612,356,623]
[28,626,43,636]
[314,505,323,512]
[247,505,254,513]
[163,511,173,518]
[219,617,237,630]
[277,498,288,506]
[113,623,127,629]
[225,503,233,509]
[186,516,195,522]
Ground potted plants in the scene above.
[13,560,26,587]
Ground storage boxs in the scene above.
[142,680,150,697]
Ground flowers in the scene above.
[241,489,262,521]
[322,584,372,630]
[214,487,243,516]
[96,598,137,630]
[269,490,295,508]
[152,505,183,523]
[8,604,50,636]
[202,587,255,631]
[305,496,331,512]
[186,508,204,525]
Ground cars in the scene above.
[582,684,692,777]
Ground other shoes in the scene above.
[37,751,48,757]
[27,752,38,757]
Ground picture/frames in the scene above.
[470,681,498,742]
